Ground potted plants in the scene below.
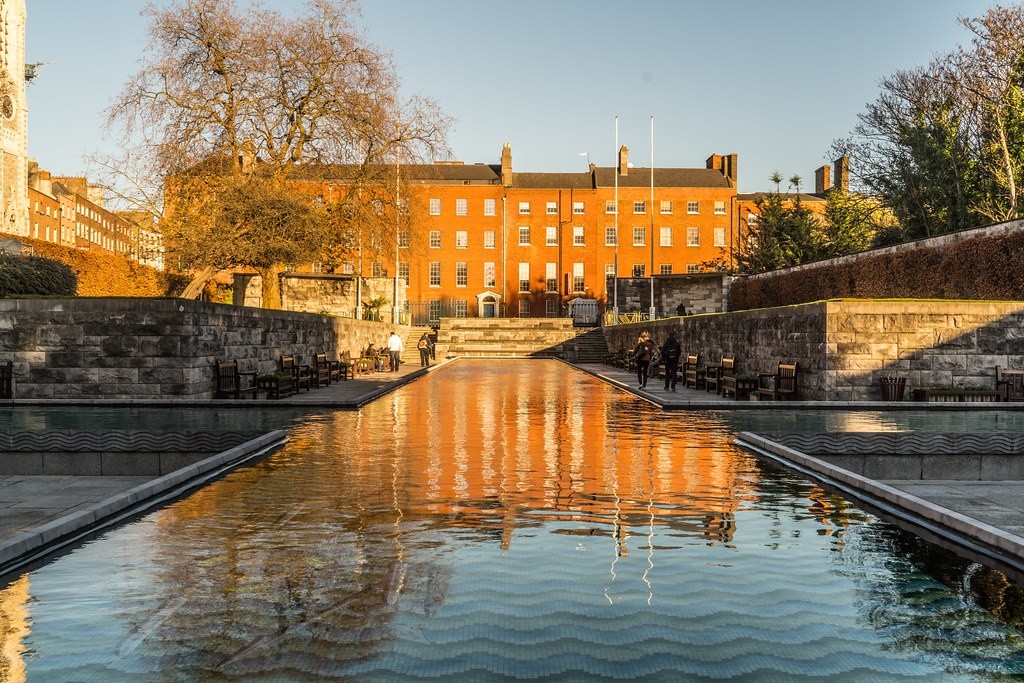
[747,391,771,401]
[698,366,712,382]
[330,361,351,375]
[735,373,755,388]
[963,386,996,402]
[300,366,325,379]
[922,384,962,402]
[261,373,288,387]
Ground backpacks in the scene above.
[666,342,677,360]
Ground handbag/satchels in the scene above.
[635,341,645,359]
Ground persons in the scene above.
[688,311,693,315]
[381,347,405,367]
[633,330,653,391]
[418,333,432,367]
[366,341,384,372]
[676,303,686,316]
[661,332,681,392]
[388,332,402,372]
[647,346,663,378]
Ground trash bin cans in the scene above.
[0,359,14,399]
[878,375,906,402]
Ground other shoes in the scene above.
[637,384,643,390]
[641,387,646,391]
[672,386,675,391]
[664,387,668,390]
[401,361,404,364]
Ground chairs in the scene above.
[281,355,310,394]
[315,352,340,384]
[215,357,259,400]
[995,365,1024,402]
[343,350,361,377]
[363,347,390,372]
[704,355,736,395]
[603,348,700,390]
[757,361,799,400]
